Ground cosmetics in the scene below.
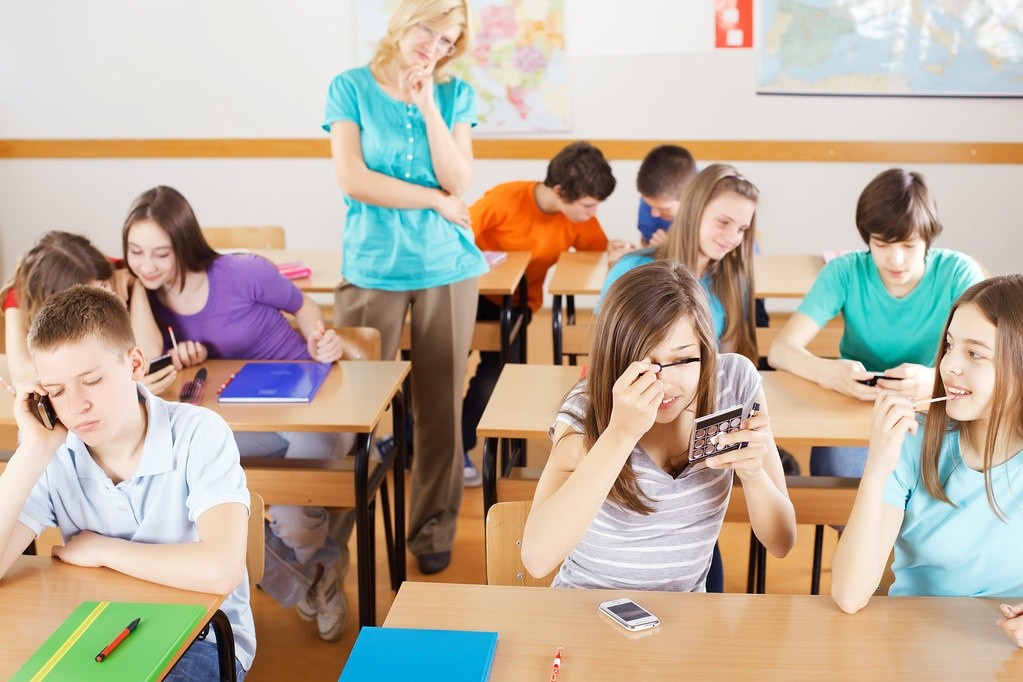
[688,405,743,465]
[741,402,760,448]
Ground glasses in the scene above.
[418,25,458,57]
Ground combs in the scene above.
[178,368,208,406]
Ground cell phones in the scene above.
[37,396,57,430]
[148,354,172,375]
[598,597,659,631]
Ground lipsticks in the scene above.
[911,395,955,409]
[167,327,178,352]
[1000,604,1017,619]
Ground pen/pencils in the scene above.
[95,617,141,662]
[0,376,16,396]
[217,371,237,395]
[550,647,563,682]
[167,326,178,350]
[1000,604,1017,619]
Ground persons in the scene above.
[321,1,492,576]
[0,286,257,682]
[520,257,796,595]
[590,162,802,480]
[122,186,351,644]
[463,140,617,489]
[828,274,1023,647]
[0,230,165,394]
[633,142,777,372]
[765,167,988,480]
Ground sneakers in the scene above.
[297,590,317,620]
[314,548,349,642]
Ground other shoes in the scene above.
[417,551,451,575]
[462,451,483,487]
[371,434,397,463]
[776,446,801,476]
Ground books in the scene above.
[337,626,499,682]
[8,602,206,682]
[218,363,331,402]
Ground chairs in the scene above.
[486,501,562,588]
[246,490,266,586]
[199,226,289,253]
[287,323,396,593]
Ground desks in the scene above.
[0,251,1022,681]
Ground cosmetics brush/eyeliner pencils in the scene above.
[637,358,702,376]
